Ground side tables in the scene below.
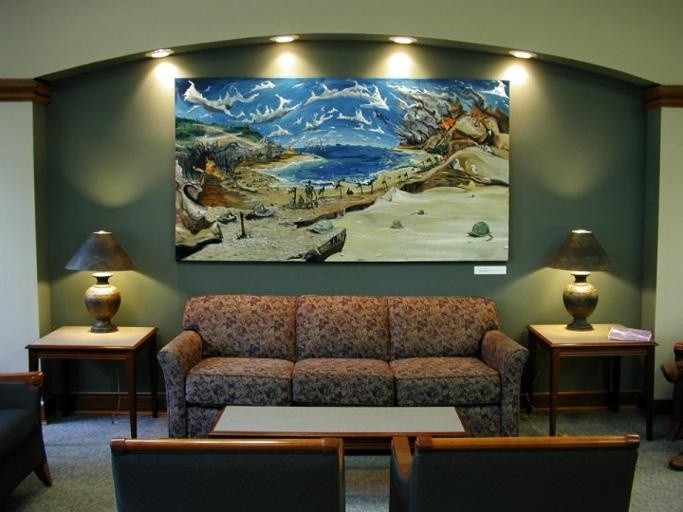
[25,326,158,441]
[526,324,658,440]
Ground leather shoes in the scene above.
[669,453,683,471]
[661,361,682,384]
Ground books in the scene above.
[608,327,652,341]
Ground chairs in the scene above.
[0,372,52,512]
[388,433,640,512]
[110,437,345,512]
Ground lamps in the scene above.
[547,230,615,329]
[66,230,139,334]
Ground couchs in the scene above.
[157,295,530,446]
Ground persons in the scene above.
[305,180,317,208]
